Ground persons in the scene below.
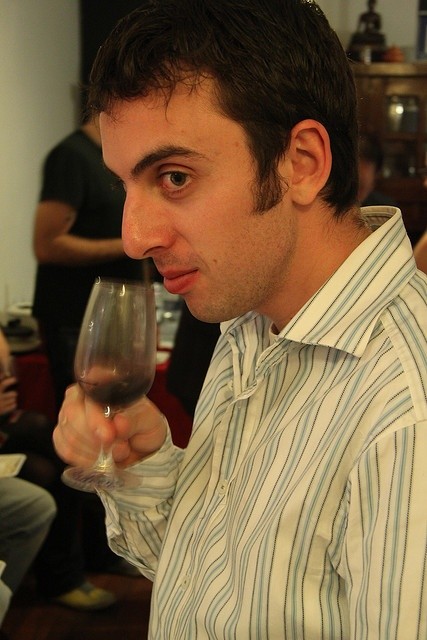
[51,1,426,636]
[0,322,137,626]
[0,477,63,640]
[357,130,385,205]
[31,108,153,611]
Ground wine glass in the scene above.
[60,276,158,495]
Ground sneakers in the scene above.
[49,580,120,613]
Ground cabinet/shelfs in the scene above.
[348,61,427,244]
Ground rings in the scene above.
[58,416,68,426]
